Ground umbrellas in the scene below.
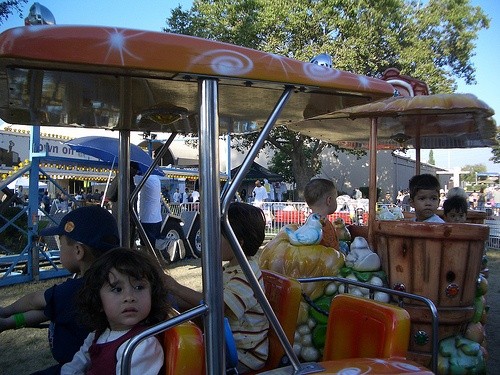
[62,136,167,208]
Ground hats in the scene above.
[41,207,120,250]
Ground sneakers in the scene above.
[166,241,178,261]
[176,239,185,259]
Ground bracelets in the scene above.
[12,313,25,330]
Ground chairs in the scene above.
[161,308,204,375]
[261,269,301,369]
[323,293,411,362]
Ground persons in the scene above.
[0,173,500,375]
